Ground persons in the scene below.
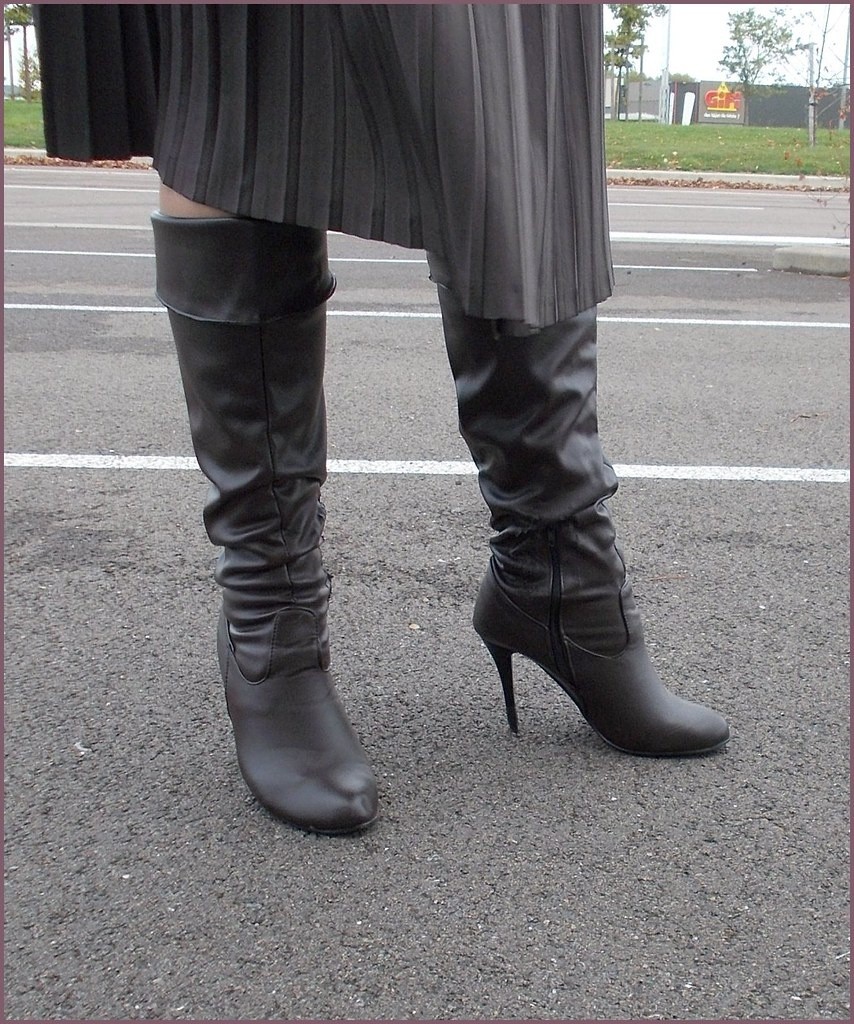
[32,4,732,834]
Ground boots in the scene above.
[152,205,378,835]
[426,249,728,757]
